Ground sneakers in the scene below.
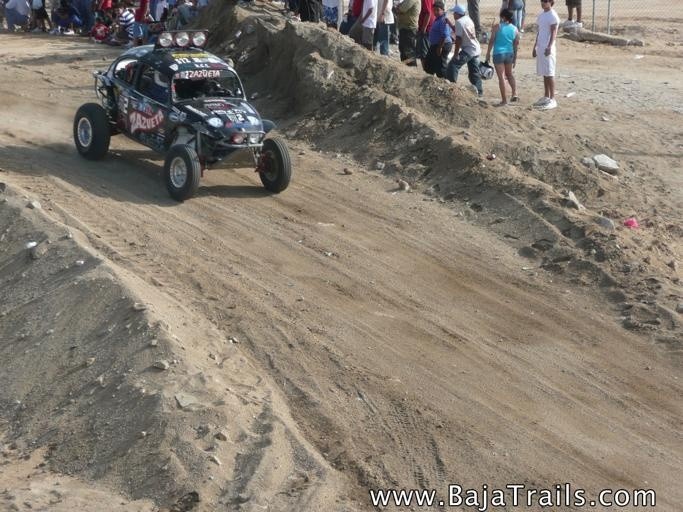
[533,96,557,108]
[30,26,74,34]
[559,19,583,29]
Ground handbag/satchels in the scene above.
[478,60,493,79]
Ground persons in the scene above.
[289,0,525,106]
[0,0,209,50]
[143,71,171,106]
[533,0,559,110]
[562,0,582,28]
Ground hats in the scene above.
[449,4,465,15]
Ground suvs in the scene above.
[67,25,290,201]
